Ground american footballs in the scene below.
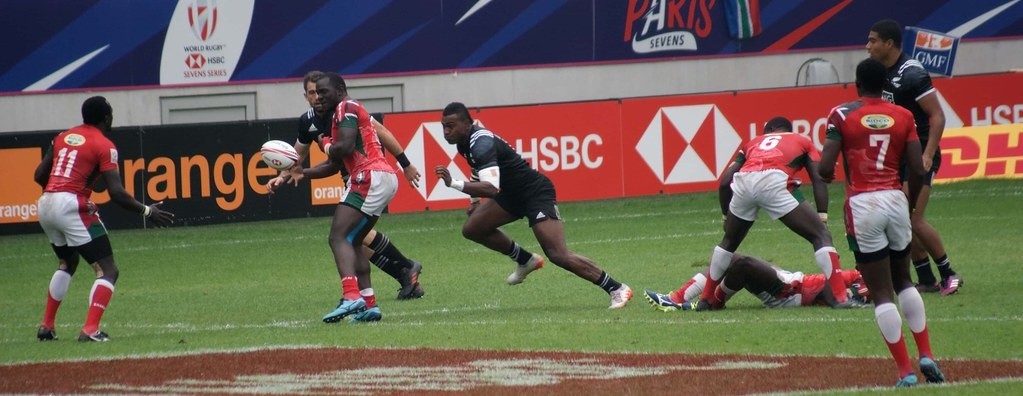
[260,140,298,171]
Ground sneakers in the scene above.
[78,329,112,343]
[38,323,59,341]
[398,259,422,300]
[914,281,941,292]
[507,254,544,286]
[695,299,726,311]
[682,296,702,311]
[895,373,918,388]
[348,305,382,323]
[939,271,963,296]
[608,283,633,311]
[921,356,945,384]
[322,298,366,323]
[833,298,873,309]
[396,267,424,299]
[643,289,683,312]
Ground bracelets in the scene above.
[818,213,828,222]
[450,178,464,192]
[396,152,410,168]
[141,205,152,217]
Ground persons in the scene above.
[266,71,425,300]
[865,20,964,296]
[817,58,944,388]
[33,96,175,342]
[435,103,634,308]
[282,73,399,324]
[694,116,863,308]
[643,253,871,311]
